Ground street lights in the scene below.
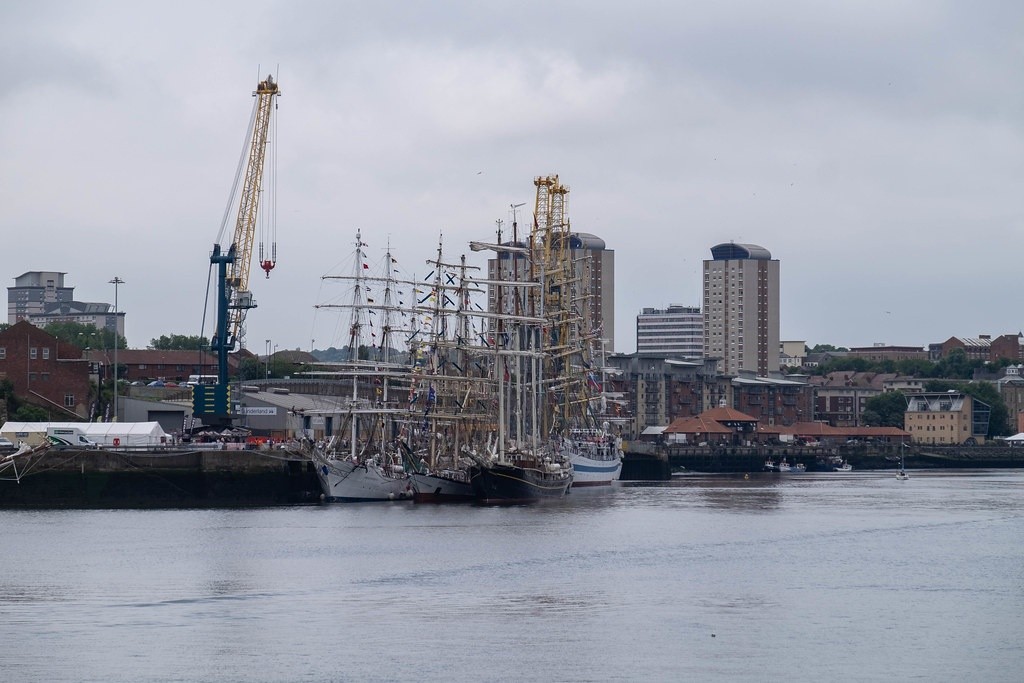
[108,276,126,424]
[273,344,278,377]
[79,332,96,359]
[265,339,271,381]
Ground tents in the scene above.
[0,421,166,452]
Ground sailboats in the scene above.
[312,174,626,503]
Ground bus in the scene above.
[187,375,218,386]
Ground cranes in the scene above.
[192,64,283,431]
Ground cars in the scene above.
[130,381,145,387]
[180,382,187,388]
[148,381,165,388]
[163,382,177,387]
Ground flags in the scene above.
[588,372,599,390]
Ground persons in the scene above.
[901,470,905,475]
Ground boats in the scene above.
[762,457,807,474]
[893,428,910,480]
[834,459,853,472]
[0,415,327,508]
